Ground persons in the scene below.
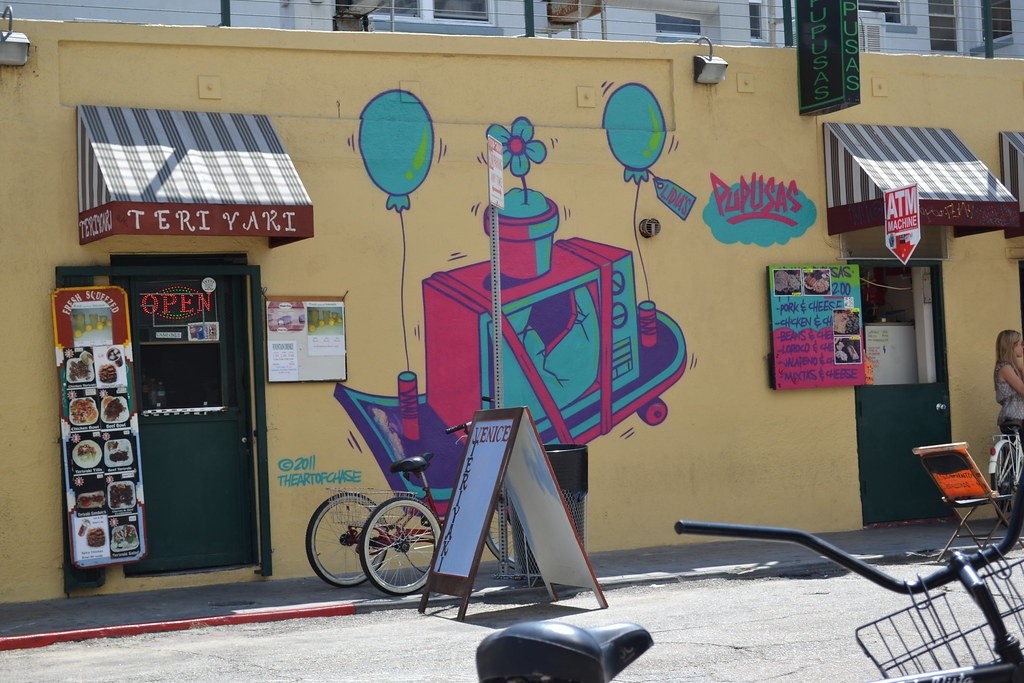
[993,330,1024,526]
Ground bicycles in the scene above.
[473,463,1024,683]
[987,420,1024,525]
[301,395,521,597]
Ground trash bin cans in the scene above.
[508,443,590,579]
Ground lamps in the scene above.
[1,28,30,66]
[695,54,729,85]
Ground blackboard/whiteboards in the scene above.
[419,406,609,622]
[765,262,869,390]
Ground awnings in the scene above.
[822,125,1019,238]
[76,106,315,250]
[998,129,1024,238]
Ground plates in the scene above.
[72,440,103,468]
[110,524,139,553]
[104,439,133,467]
[107,481,136,510]
[66,358,95,383]
[101,396,130,424]
[69,397,99,425]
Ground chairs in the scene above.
[913,440,1024,563]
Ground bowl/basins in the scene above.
[106,347,121,362]
